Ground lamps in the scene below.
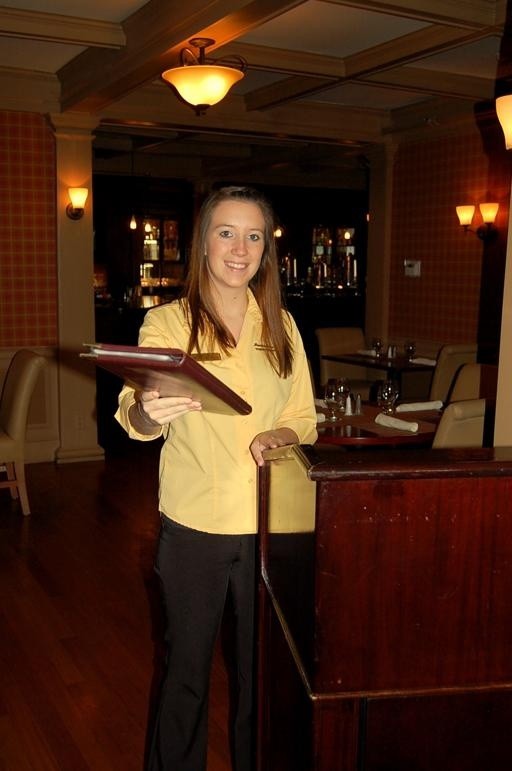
[162,38,245,116]
[66,187,89,220]
[456,205,476,231]
[495,94,512,149]
[479,203,499,228]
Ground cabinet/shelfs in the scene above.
[304,217,367,295]
[133,214,185,294]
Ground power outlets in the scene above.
[404,260,421,277]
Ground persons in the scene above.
[115,185,320,770]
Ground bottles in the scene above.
[345,392,362,417]
[387,344,397,360]
[280,231,355,298]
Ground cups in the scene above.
[329,378,349,414]
[376,381,400,418]
[406,342,416,363]
[372,338,383,359]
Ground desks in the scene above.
[320,353,436,404]
[314,399,444,454]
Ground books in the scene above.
[78,341,251,417]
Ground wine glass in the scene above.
[324,385,343,424]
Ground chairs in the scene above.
[428,344,477,402]
[451,364,482,402]
[431,399,486,449]
[0,349,48,515]
[316,326,375,400]
